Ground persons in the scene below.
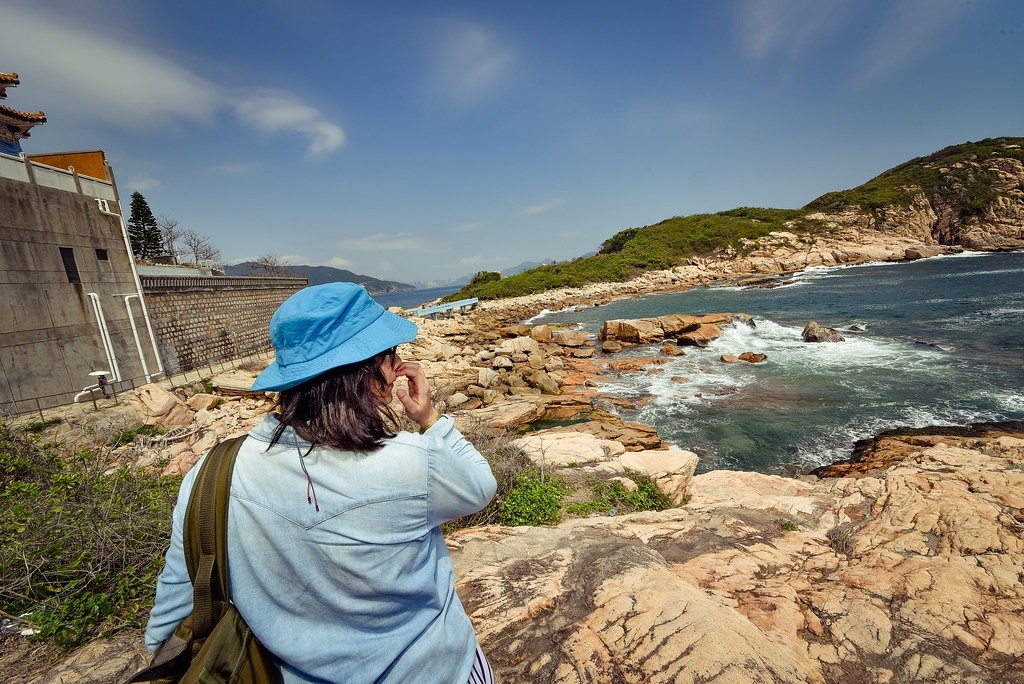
[144,282,500,683]
[97,375,110,399]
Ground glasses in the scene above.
[379,345,397,367]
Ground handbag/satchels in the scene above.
[122,434,283,684]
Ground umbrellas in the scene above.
[88,371,110,375]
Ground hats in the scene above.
[250,281,418,392]
